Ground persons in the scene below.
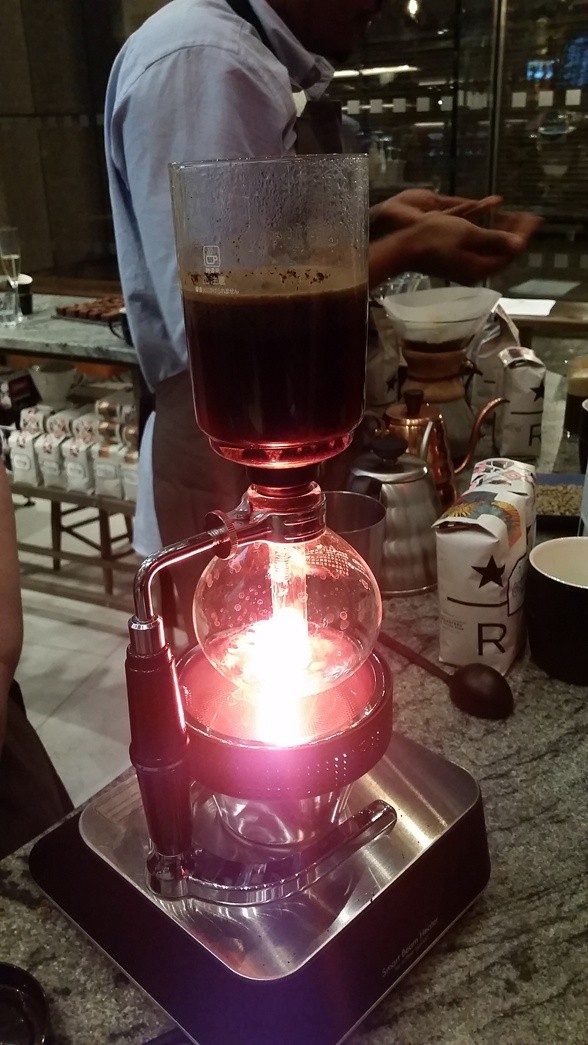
[0,465,76,864]
[104,0,539,659]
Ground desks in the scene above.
[493,298,588,356]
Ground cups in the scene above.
[527,536,588,688]
[578,399,588,475]
[107,306,134,347]
[563,353,588,442]
[29,362,86,408]
[0,292,16,329]
[17,272,33,316]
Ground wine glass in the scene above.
[0,226,22,324]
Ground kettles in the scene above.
[344,421,445,598]
[359,389,511,519]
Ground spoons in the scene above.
[377,631,515,721]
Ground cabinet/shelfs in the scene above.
[0,291,144,615]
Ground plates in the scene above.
[0,962,55,1045]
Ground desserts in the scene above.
[56,297,124,322]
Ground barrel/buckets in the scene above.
[322,491,387,593]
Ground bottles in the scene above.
[399,347,475,468]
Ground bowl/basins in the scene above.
[385,314,490,353]
